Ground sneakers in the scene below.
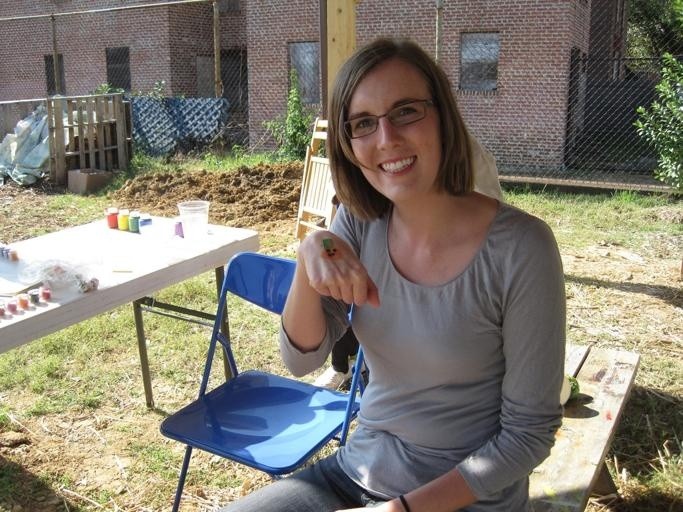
[311,357,365,390]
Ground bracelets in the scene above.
[397,492,411,508]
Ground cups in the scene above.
[177,200,211,242]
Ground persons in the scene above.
[214,35,566,512]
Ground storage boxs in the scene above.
[67,169,112,197]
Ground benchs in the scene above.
[523,345,642,511]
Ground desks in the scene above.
[0,209,262,414]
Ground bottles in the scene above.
[103,207,142,233]
[0,286,51,316]
[0,244,19,262]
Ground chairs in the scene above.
[158,252,366,511]
[295,116,335,240]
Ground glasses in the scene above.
[342,98,436,139]
